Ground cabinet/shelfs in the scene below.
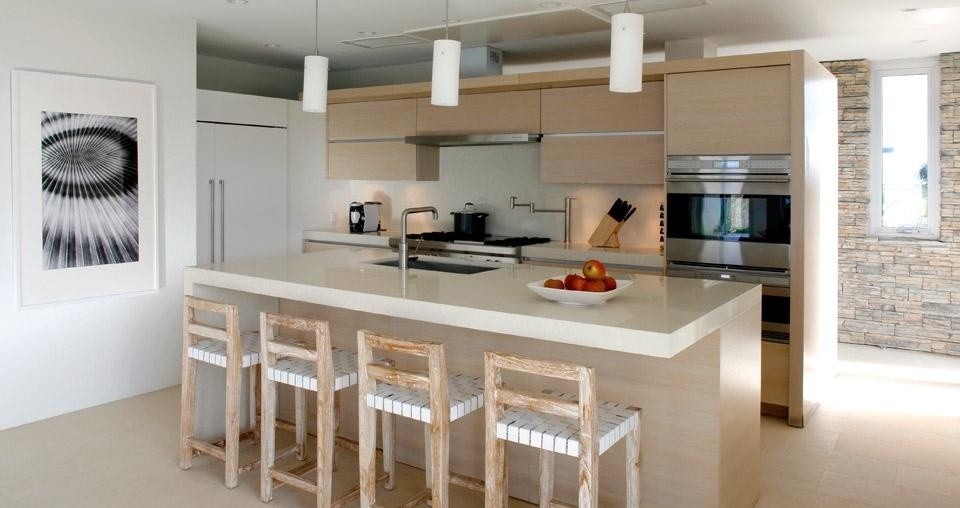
[761,338,793,420]
[300,241,349,248]
[408,83,541,133]
[540,73,665,192]
[323,91,441,183]
[661,56,795,159]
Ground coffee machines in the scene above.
[349,201,381,233]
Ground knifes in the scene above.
[607,196,638,224]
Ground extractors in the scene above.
[403,133,540,147]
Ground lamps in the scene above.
[430,2,464,109]
[301,1,329,114]
[602,1,644,93]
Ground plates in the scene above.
[525,273,635,304]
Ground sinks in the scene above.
[366,251,506,276]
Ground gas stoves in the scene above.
[389,230,551,264]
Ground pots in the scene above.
[451,202,489,238]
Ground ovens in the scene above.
[664,180,791,345]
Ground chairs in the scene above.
[258,309,396,507]
[481,348,646,507]
[354,327,498,506]
[177,291,312,488]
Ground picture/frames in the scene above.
[7,63,163,308]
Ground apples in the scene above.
[545,278,564,288]
[565,259,617,291]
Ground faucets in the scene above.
[395,205,440,271]
